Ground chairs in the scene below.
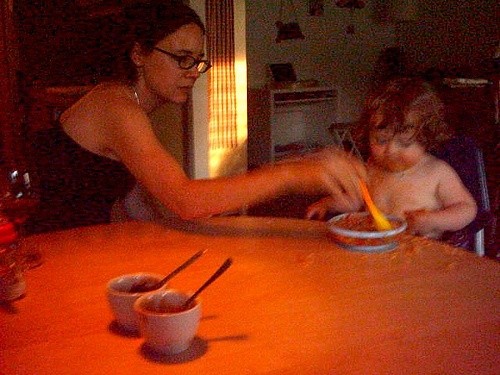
[343,138,500,262]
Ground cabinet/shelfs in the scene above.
[248,85,339,171]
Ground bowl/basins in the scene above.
[134,290,203,355]
[329,211,409,253]
[106,274,170,333]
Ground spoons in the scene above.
[349,164,393,234]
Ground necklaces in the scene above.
[132,85,140,105]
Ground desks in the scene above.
[0,215,500,375]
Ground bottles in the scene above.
[0,214,27,303]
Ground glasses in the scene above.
[151,46,212,74]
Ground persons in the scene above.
[304,78,478,241]
[24,0,370,235]
